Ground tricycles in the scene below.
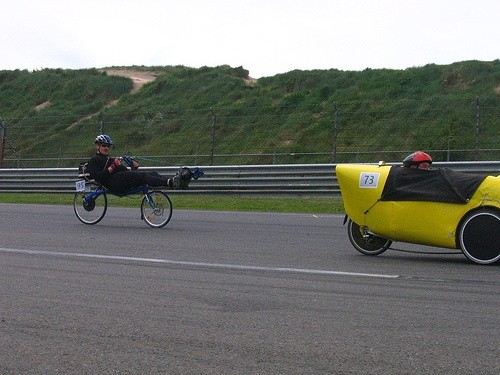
[334,162,500,266]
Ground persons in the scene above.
[86,134,191,197]
[402,150,433,169]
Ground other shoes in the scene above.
[173,167,193,190]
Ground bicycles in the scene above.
[71,155,203,230]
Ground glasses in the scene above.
[101,145,110,148]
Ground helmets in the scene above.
[95,135,113,145]
[403,151,432,166]
